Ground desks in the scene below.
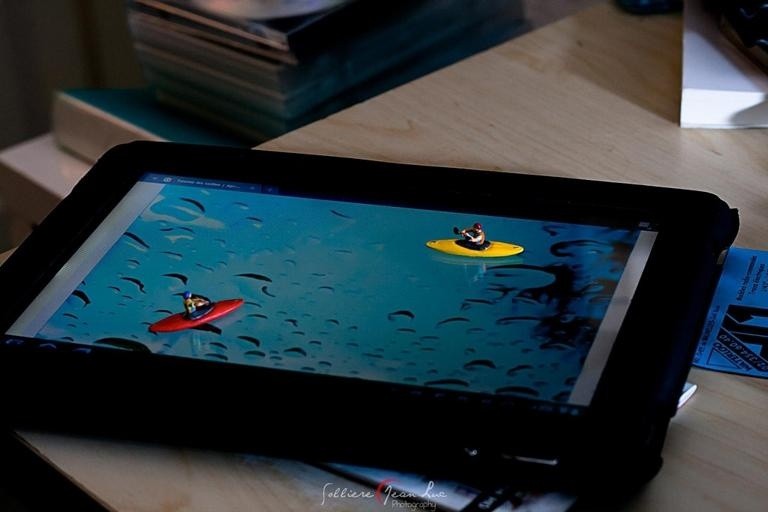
[0,2,767,509]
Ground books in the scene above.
[682,0,768,131]
[129,0,528,144]
[314,381,699,511]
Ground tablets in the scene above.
[0,141,740,496]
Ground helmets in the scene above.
[182,291,192,299]
[473,223,481,229]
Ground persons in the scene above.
[183,289,211,317]
[461,220,485,247]
